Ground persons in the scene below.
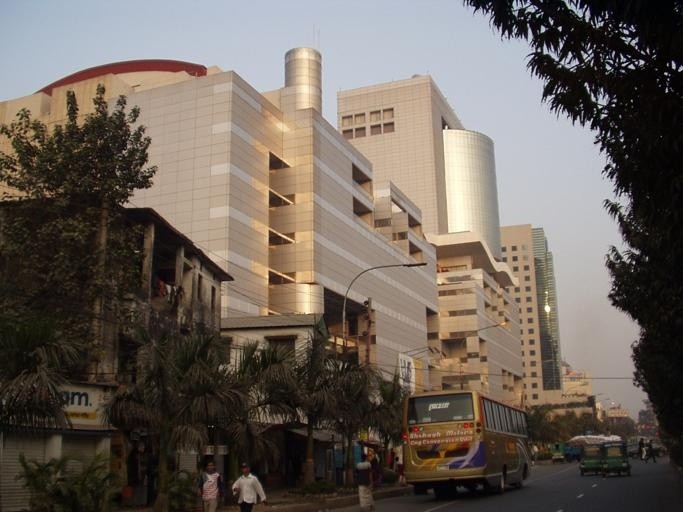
[387,446,395,471]
[397,463,406,486]
[644,439,657,465]
[366,447,383,490]
[637,437,645,465]
[528,442,538,474]
[194,461,224,511]
[392,457,400,474]
[231,462,269,511]
[353,453,376,511]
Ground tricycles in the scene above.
[550,438,632,478]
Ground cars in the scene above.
[626,443,667,460]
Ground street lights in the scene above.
[519,359,616,417]
[343,262,427,488]
[460,321,507,390]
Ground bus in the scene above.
[402,389,536,498]
